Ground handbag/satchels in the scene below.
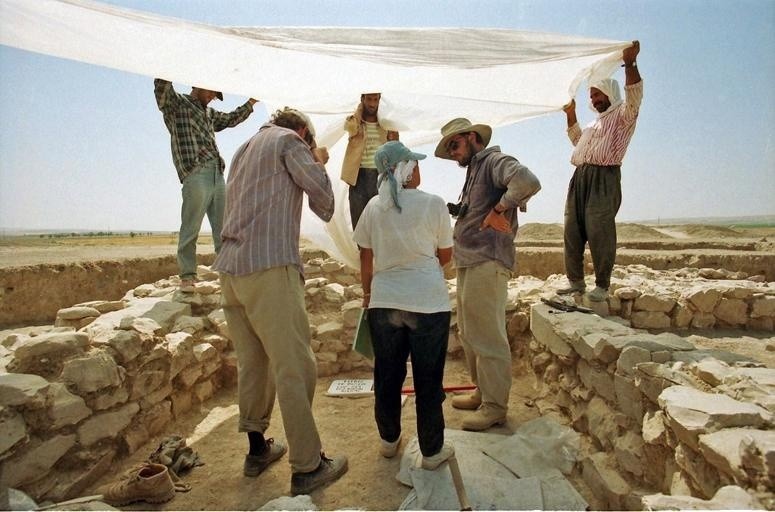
[492,205,505,216]
[361,291,371,299]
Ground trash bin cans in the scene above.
[620,60,637,70]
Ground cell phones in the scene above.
[448,199,467,220]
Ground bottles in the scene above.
[435,118,492,161]
[375,140,426,170]
[284,108,316,147]
[192,87,222,101]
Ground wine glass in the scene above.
[505,223,508,227]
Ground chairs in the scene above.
[587,286,609,301]
[243,438,287,477]
[557,279,588,295]
[381,433,403,458]
[422,443,455,470]
[452,387,482,410]
[461,402,508,431]
[94,463,175,504]
[180,279,193,293]
[291,455,348,495]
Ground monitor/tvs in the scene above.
[351,306,375,361]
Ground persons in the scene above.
[434,118,543,433]
[349,139,457,473]
[153,77,260,294]
[555,40,644,303]
[340,91,400,253]
[208,106,351,498]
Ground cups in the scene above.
[443,136,467,153]
